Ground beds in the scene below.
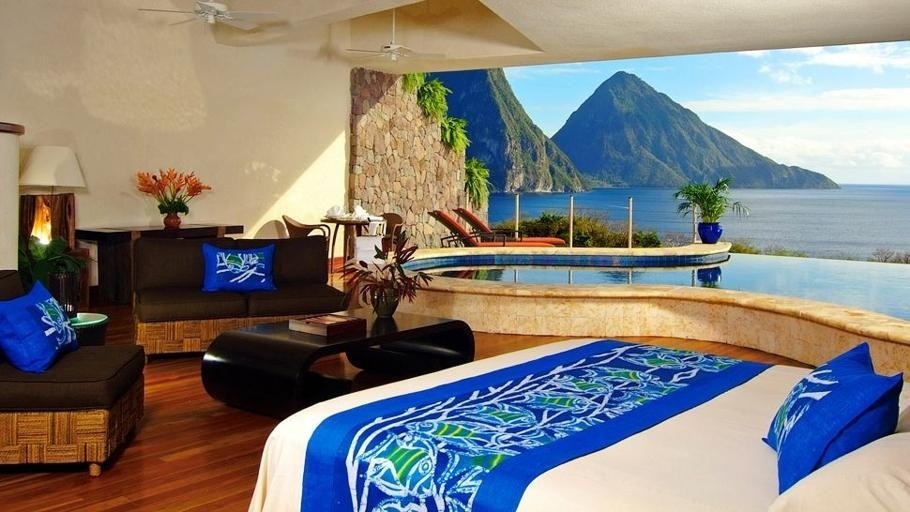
[246,338,906,510]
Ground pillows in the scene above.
[763,429,910,512]
[890,378,909,433]
[760,340,905,496]
[201,242,277,292]
[0,280,80,373]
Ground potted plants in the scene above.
[22,236,89,320]
[696,265,724,289]
[335,230,433,319]
[672,175,751,245]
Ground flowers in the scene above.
[136,169,213,214]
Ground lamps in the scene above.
[19,144,88,249]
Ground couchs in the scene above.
[1,271,147,476]
[131,236,352,356]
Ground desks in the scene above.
[74,225,246,298]
[314,215,382,277]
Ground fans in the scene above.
[343,1,445,67]
[134,1,279,30]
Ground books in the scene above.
[288,313,366,337]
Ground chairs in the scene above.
[378,212,404,250]
[281,214,330,273]
[430,210,555,248]
[452,206,568,247]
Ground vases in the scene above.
[162,211,181,226]
